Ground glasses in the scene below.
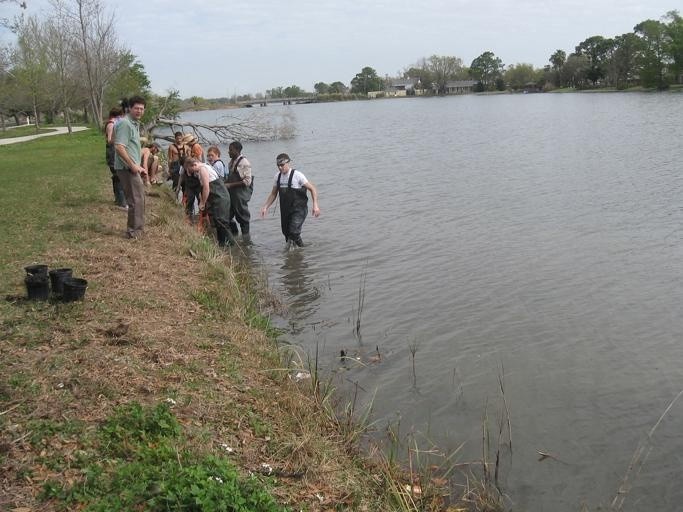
[276,163,285,167]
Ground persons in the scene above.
[259,152,319,250]
[104,107,129,211]
[113,96,148,242]
[110,97,148,145]
[140,141,164,188]
[167,131,253,245]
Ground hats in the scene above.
[181,134,194,145]
[152,141,161,152]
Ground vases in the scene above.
[24,264,89,303]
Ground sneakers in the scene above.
[126,230,136,238]
[113,204,129,212]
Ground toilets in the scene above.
[23,265,88,302]
